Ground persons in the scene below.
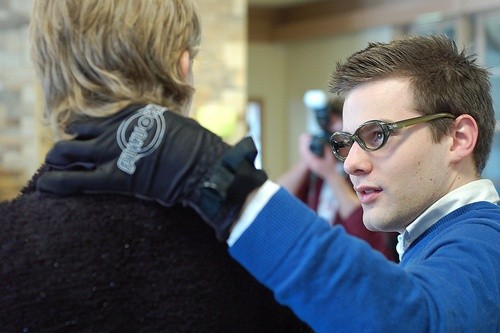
[0,0,310,333]
[36,32,500,333]
[274,98,395,263]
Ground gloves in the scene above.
[36,103,268,243]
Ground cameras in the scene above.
[309,109,335,158]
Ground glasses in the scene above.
[330,112,456,160]
